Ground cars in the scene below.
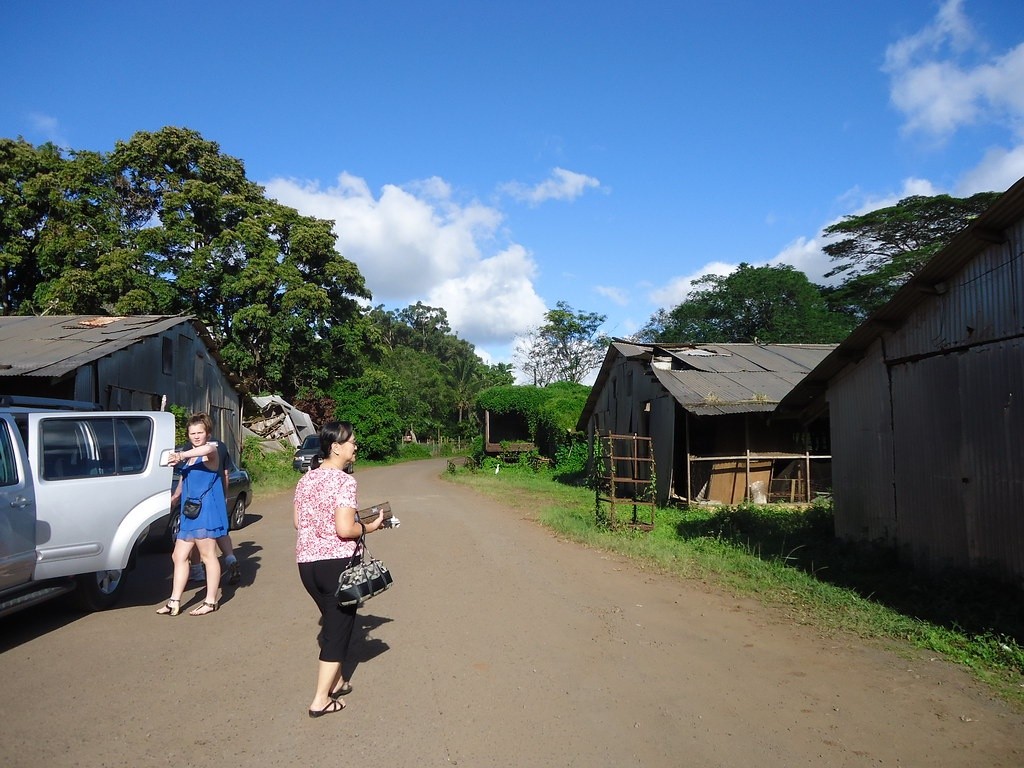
[292,433,354,475]
[145,437,253,552]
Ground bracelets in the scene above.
[177,451,182,461]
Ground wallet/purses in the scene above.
[356,502,392,524]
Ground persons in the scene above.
[294,422,385,718]
[156,412,240,615]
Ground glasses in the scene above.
[340,440,356,445]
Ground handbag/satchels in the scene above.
[183,497,202,519]
[335,524,394,606]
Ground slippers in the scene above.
[310,682,352,717]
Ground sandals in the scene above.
[156,599,180,615]
[190,602,218,615]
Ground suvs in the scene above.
[0,395,177,627]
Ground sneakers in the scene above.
[226,555,240,586]
[189,564,205,581]
